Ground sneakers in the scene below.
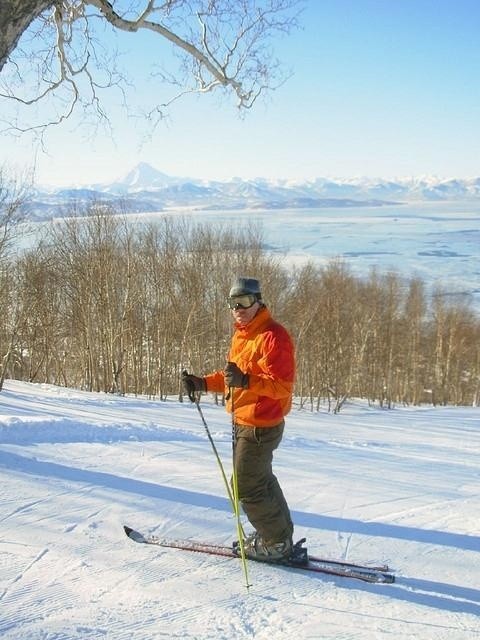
[232,531,293,558]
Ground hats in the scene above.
[228,277,261,296]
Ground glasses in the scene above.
[227,295,261,309]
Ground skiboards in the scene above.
[124,526,395,583]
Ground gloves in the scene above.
[224,361,248,388]
[182,375,207,393]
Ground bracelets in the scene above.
[242,375,248,388]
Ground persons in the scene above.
[182,278,296,561]
